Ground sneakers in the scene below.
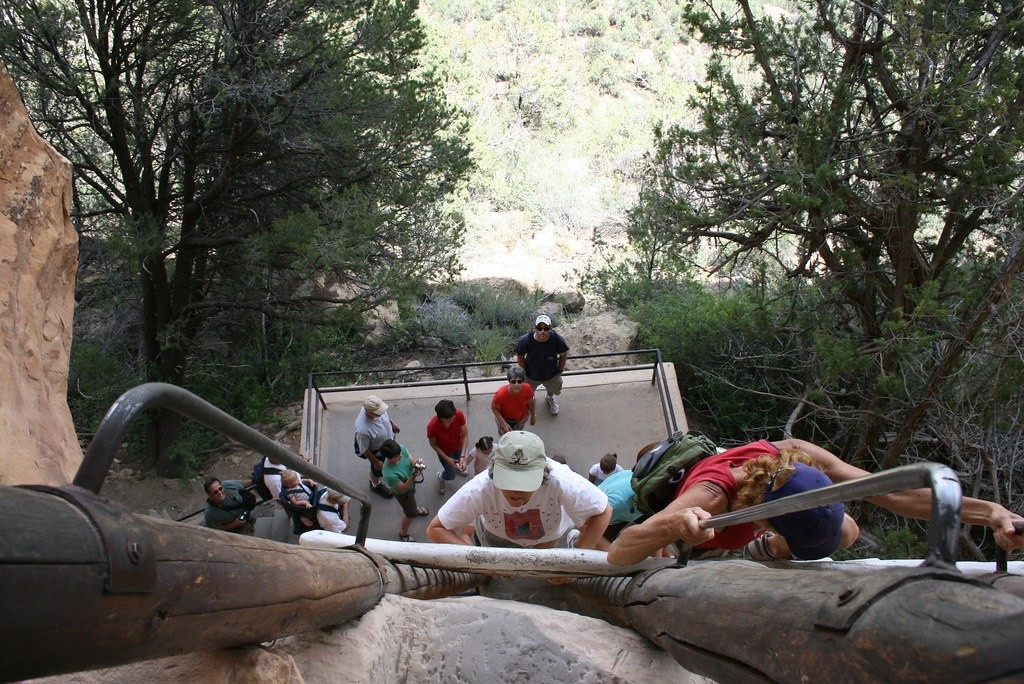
[368,480,394,499]
[545,397,559,415]
[745,531,778,562]
[300,514,313,527]
[566,528,582,549]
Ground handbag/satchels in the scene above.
[238,489,256,510]
[354,436,367,460]
[630,430,717,518]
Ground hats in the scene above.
[535,315,551,326]
[492,430,546,493]
[326,486,352,504]
[363,395,389,416]
[766,459,844,561]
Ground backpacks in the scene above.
[252,455,283,501]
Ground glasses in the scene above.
[214,486,223,495]
[535,325,551,331]
[510,379,523,384]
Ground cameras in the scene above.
[415,461,426,469]
[240,511,257,525]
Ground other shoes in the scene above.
[456,461,468,477]
[436,472,445,496]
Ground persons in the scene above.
[459,436,497,476]
[551,454,579,474]
[607,438,1024,568]
[515,315,570,415]
[354,394,400,499]
[317,486,352,534]
[380,439,429,542]
[427,398,467,495]
[251,440,312,523]
[282,469,316,526]
[426,430,613,585]
[589,454,676,559]
[203,477,252,538]
[491,366,536,437]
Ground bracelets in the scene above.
[460,456,465,458]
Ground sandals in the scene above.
[415,506,429,515]
[399,530,414,542]
